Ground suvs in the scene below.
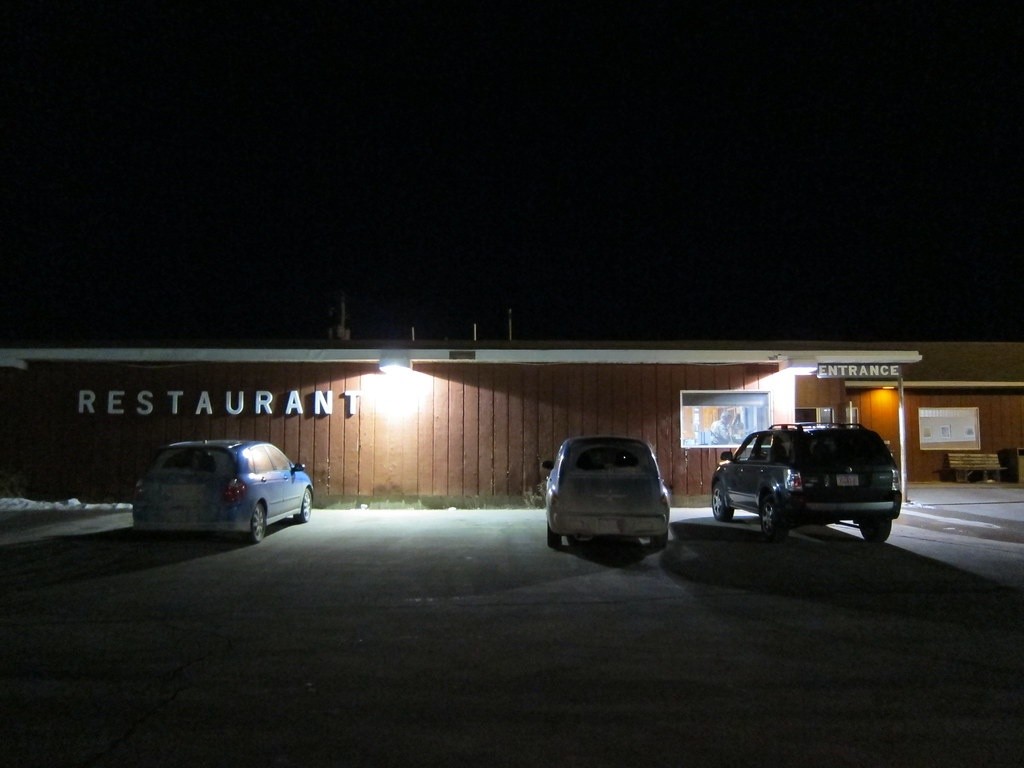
[710,422,904,544]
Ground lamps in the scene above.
[779,360,817,372]
[0,358,28,370]
[379,359,413,372]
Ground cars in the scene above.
[130,437,315,544]
[539,435,671,550]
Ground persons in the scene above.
[710,411,731,444]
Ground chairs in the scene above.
[771,445,786,457]
[813,443,830,454]
[200,455,216,472]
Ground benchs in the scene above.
[948,453,1007,482]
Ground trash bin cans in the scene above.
[1010,447,1024,483]
